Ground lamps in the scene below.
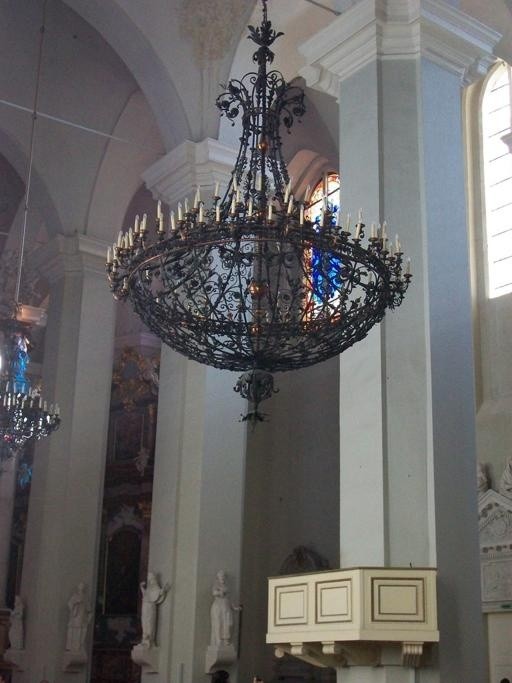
[0,0,62,454]
[98,2,413,435]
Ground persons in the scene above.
[476,457,512,498]
[210,570,242,643]
[8,594,25,650]
[66,582,93,651]
[139,571,167,640]
